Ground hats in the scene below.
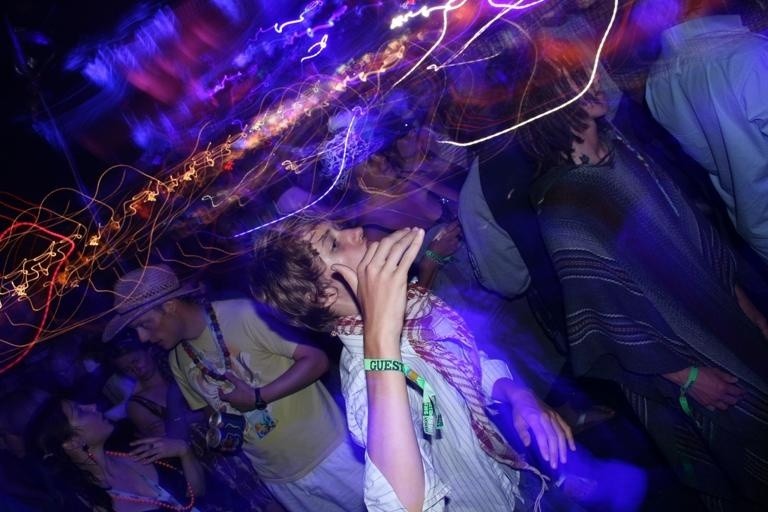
[102,264,201,343]
[320,125,405,177]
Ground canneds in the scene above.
[209,411,245,431]
[205,427,244,453]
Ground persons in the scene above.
[1,2,767,512]
